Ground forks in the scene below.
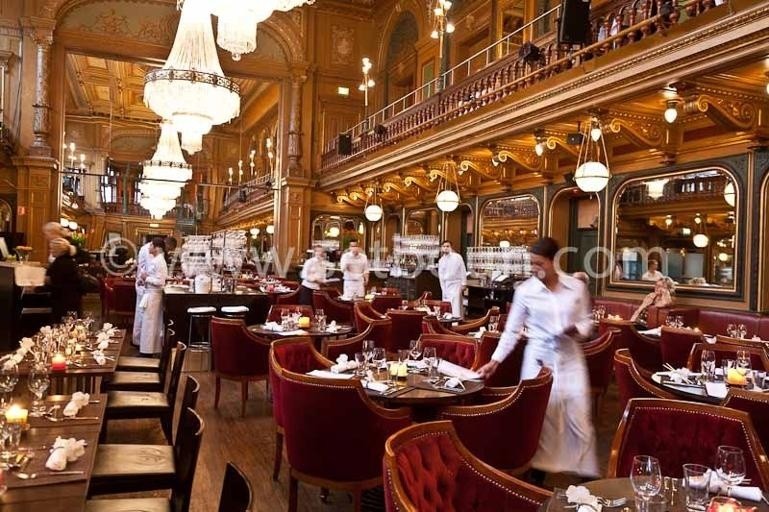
[557,489,627,508]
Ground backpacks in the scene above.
[56,258,101,294]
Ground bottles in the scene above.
[465,245,533,278]
[392,232,440,256]
[179,227,248,276]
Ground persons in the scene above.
[41,222,77,263]
[138,239,167,356]
[642,260,664,281]
[438,239,470,327]
[339,236,370,298]
[476,238,603,487]
[43,238,84,324]
[299,245,328,292]
[131,236,178,347]
[72,168,87,206]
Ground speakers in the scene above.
[338,133,351,155]
[559,0,589,44]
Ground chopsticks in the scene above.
[663,362,693,385]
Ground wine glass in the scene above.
[354,336,441,389]
[700,349,716,382]
[280,307,326,332]
[590,305,606,323]
[628,445,748,512]
[1,308,93,471]
[734,349,752,390]
[665,314,684,328]
[726,323,747,340]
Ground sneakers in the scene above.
[130,341,139,347]
[136,353,153,358]
